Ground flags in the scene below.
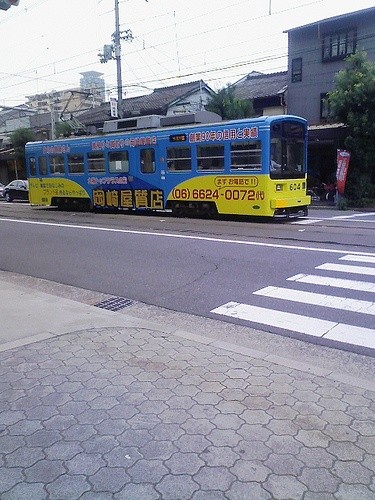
[336,149,350,193]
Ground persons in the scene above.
[270,153,286,173]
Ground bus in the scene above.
[24,89,312,224]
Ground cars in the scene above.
[3,180,29,202]
